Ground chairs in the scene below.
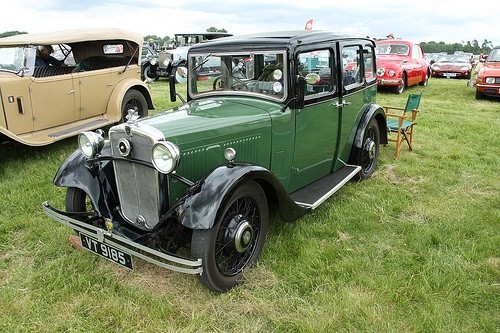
[383,91,422,160]
[253,64,283,95]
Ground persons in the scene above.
[478,52,485,70]
[35,44,54,65]
[146,39,156,49]
[387,34,396,53]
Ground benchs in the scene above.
[29,56,134,78]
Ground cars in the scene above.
[344,39,430,95]
[430,55,475,80]
[141,32,239,85]
[0,27,156,147]
[42,30,388,295]
[423,51,486,66]
[236,49,350,80]
[475,46,500,101]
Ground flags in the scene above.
[305,20,313,30]
